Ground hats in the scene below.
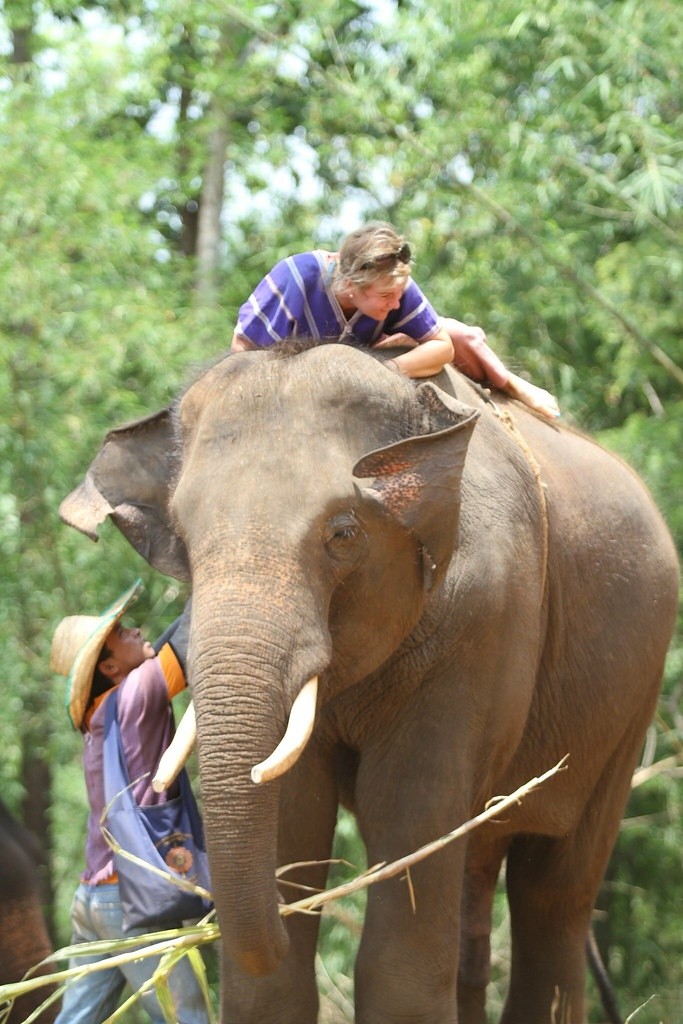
[50,578,147,732]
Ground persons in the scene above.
[49,580,216,1024]
[232,226,561,421]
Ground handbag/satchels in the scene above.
[105,690,215,933]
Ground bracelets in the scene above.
[389,358,399,371]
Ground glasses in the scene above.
[360,243,411,274]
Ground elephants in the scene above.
[1,328,682,1024]
